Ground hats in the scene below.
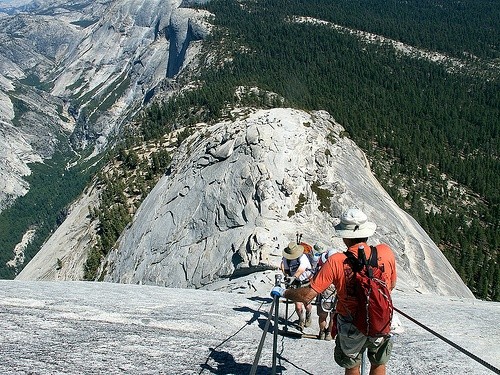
[334,209,377,239]
[314,242,327,254]
[282,242,304,260]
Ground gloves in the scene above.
[270,286,286,298]
[286,276,296,285]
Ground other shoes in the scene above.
[325,330,332,340]
[319,329,326,340]
[297,320,304,330]
[305,318,312,327]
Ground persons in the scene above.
[270,209,397,375]
[281,243,313,331]
[313,250,338,340]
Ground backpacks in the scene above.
[343,246,394,338]
[287,242,318,273]
[320,283,338,312]
